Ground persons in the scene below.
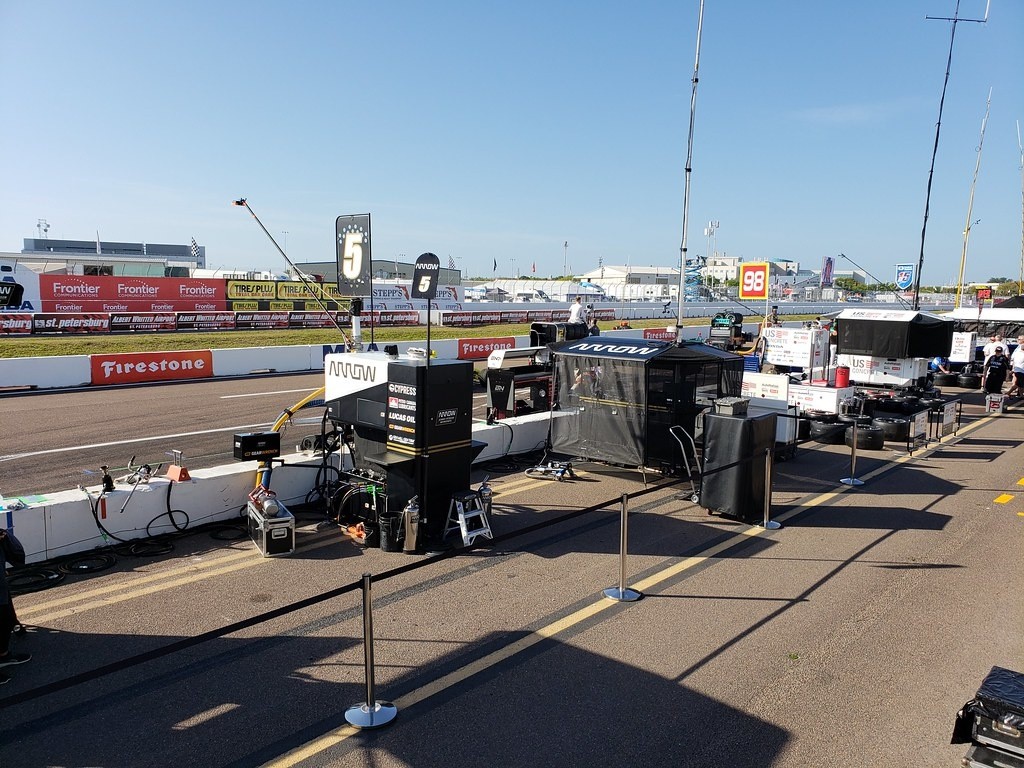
[985,335,1009,381]
[770,315,839,366]
[568,296,584,324]
[1010,335,1024,397]
[931,356,950,373]
[1005,345,1024,397]
[983,336,995,379]
[982,346,1011,395]
[589,319,599,336]
[1,530,32,684]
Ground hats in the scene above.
[995,346,1003,352]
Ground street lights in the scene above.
[231,196,363,353]
[704,228,714,286]
[510,258,516,279]
[281,231,290,272]
[708,220,719,289]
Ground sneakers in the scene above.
[2,651,32,667]
[1,674,12,685]
